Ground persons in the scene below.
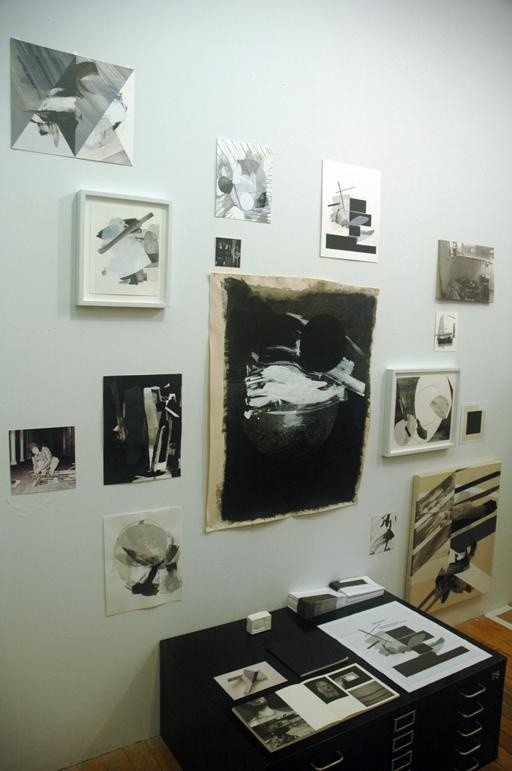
[30,440,59,478]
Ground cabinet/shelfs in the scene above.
[161,588,507,771]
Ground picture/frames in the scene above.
[75,191,174,308]
[381,367,463,458]
